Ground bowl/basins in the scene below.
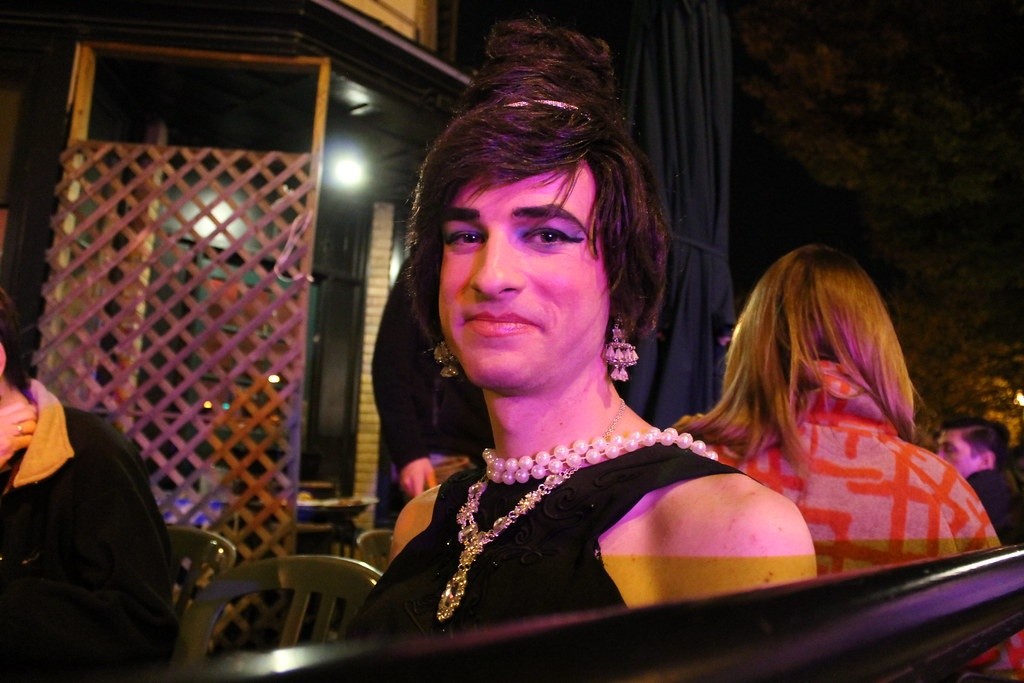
[298,498,378,521]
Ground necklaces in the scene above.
[439,398,624,622]
[484,428,721,486]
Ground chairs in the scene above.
[161,525,393,667]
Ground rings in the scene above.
[17,424,23,432]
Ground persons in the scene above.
[336,22,814,643]
[0,288,174,683]
[667,245,1024,683]
[371,234,492,531]
[936,416,1024,543]
